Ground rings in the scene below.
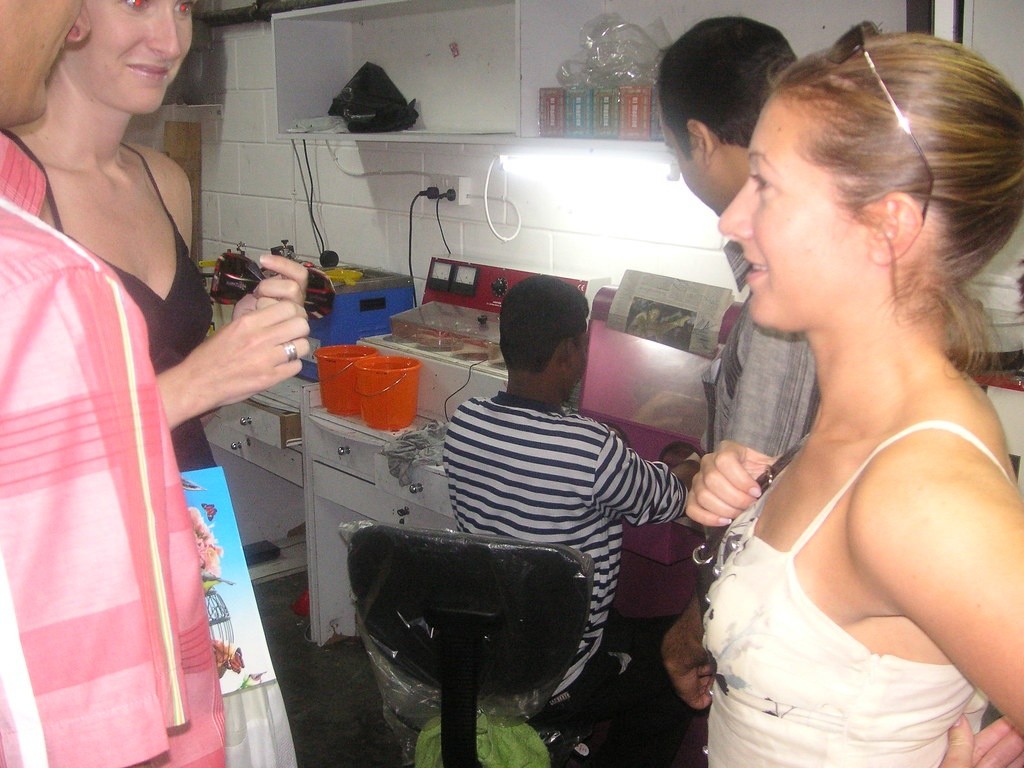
[282,341,298,362]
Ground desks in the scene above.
[196,377,458,647]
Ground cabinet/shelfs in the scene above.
[270,1,933,151]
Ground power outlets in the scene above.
[426,174,471,206]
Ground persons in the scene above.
[441,274,701,768]
[656,15,823,768]
[684,30,1024,768]
[1,0,311,768]
[626,301,696,344]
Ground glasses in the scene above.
[209,252,334,320]
[830,20,934,226]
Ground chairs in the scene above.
[347,525,599,768]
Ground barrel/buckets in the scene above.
[311,344,380,417]
[354,355,422,430]
